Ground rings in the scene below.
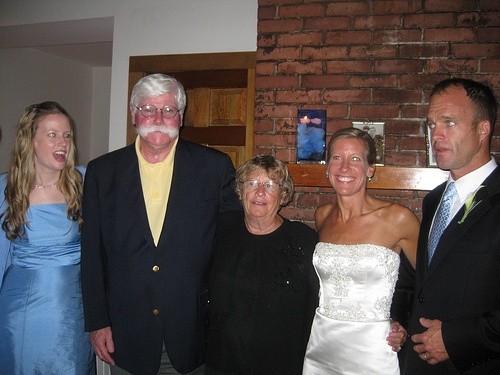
[95,349,101,355]
[398,347,401,350]
[424,352,433,360]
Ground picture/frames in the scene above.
[353,121,386,166]
[296,109,329,165]
[427,121,438,167]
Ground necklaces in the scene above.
[35,179,59,189]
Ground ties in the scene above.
[427,182,456,266]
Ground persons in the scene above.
[0,102,91,375]
[389,78,500,375]
[301,128,421,375]
[208,156,408,375]
[66,72,245,375]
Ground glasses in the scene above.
[135,104,181,119]
[240,181,283,194]
